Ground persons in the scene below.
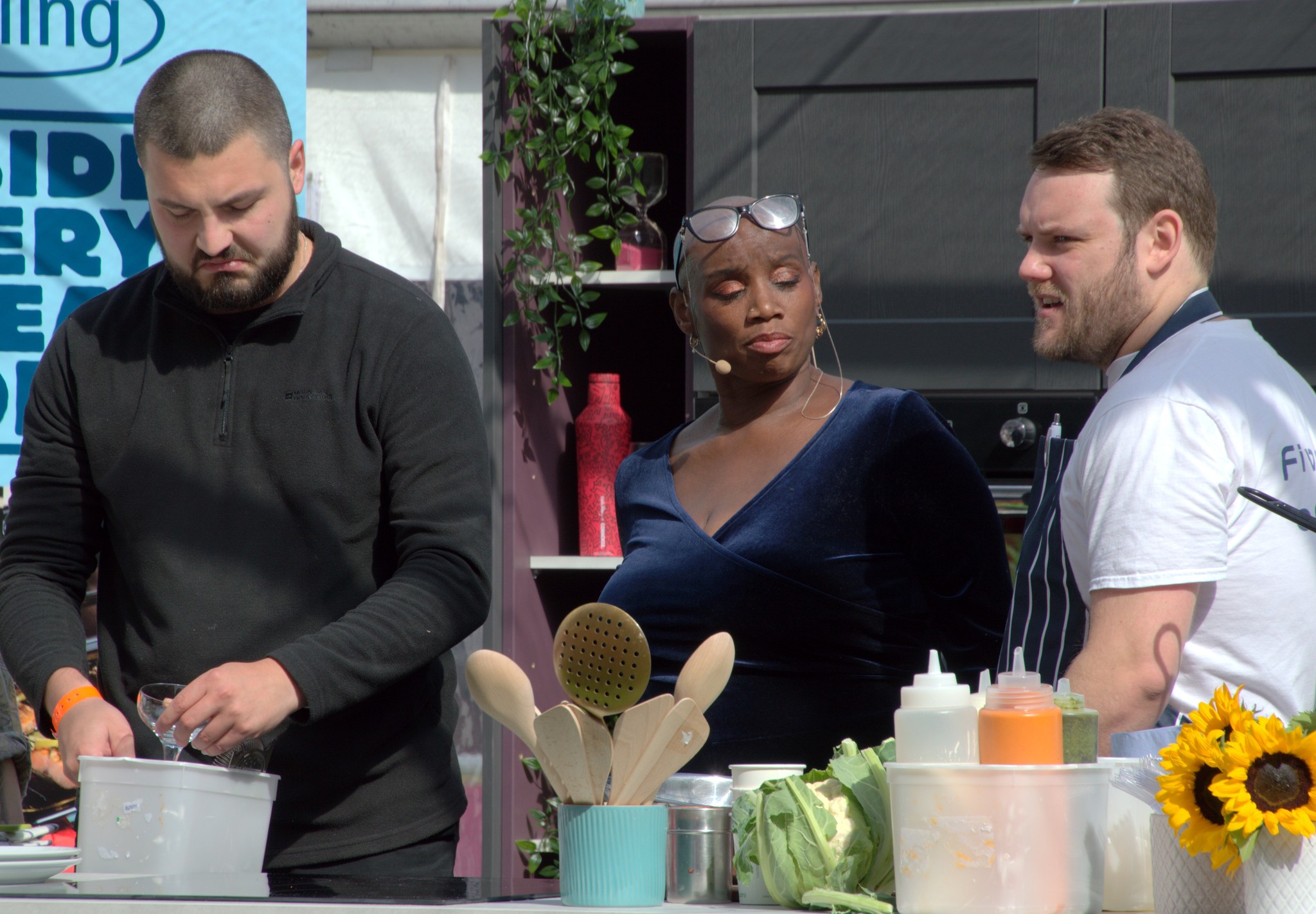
[1,46,506,913]
[994,110,1316,757]
[599,184,1017,775]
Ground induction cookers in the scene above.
[0,869,561,905]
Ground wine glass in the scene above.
[136,683,216,761]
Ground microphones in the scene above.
[689,332,731,375]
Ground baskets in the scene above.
[1242,821,1316,914]
[1149,813,1245,914]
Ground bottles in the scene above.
[977,646,1064,764]
[666,804,735,904]
[893,647,980,766]
[573,373,633,555]
[966,667,993,708]
[1051,677,1101,764]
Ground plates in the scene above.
[0,846,84,885]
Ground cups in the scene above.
[728,763,807,904]
[558,804,668,907]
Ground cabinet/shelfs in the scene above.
[520,265,686,572]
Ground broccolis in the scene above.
[728,738,896,914]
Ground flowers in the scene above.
[1151,674,1316,879]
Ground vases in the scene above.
[1148,812,1248,914]
[1238,817,1316,914]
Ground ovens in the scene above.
[888,382,1107,596]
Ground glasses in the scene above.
[675,194,809,290]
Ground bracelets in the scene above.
[52,685,103,732]
